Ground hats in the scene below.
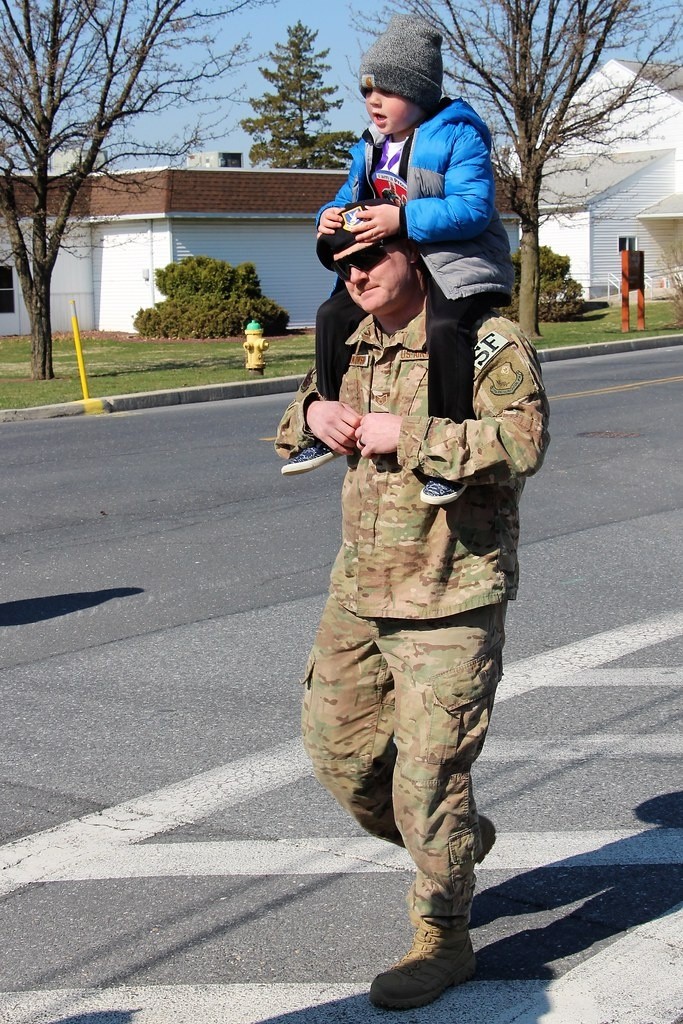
[359,13,443,110]
[316,198,413,272]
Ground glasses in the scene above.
[331,236,403,280]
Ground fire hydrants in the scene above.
[242,320,269,375]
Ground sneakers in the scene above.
[473,815,496,871]
[421,477,467,505]
[370,920,476,1011]
[279,441,342,477]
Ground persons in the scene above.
[273,199,550,1009]
[280,9,515,503]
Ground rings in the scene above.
[359,438,366,447]
